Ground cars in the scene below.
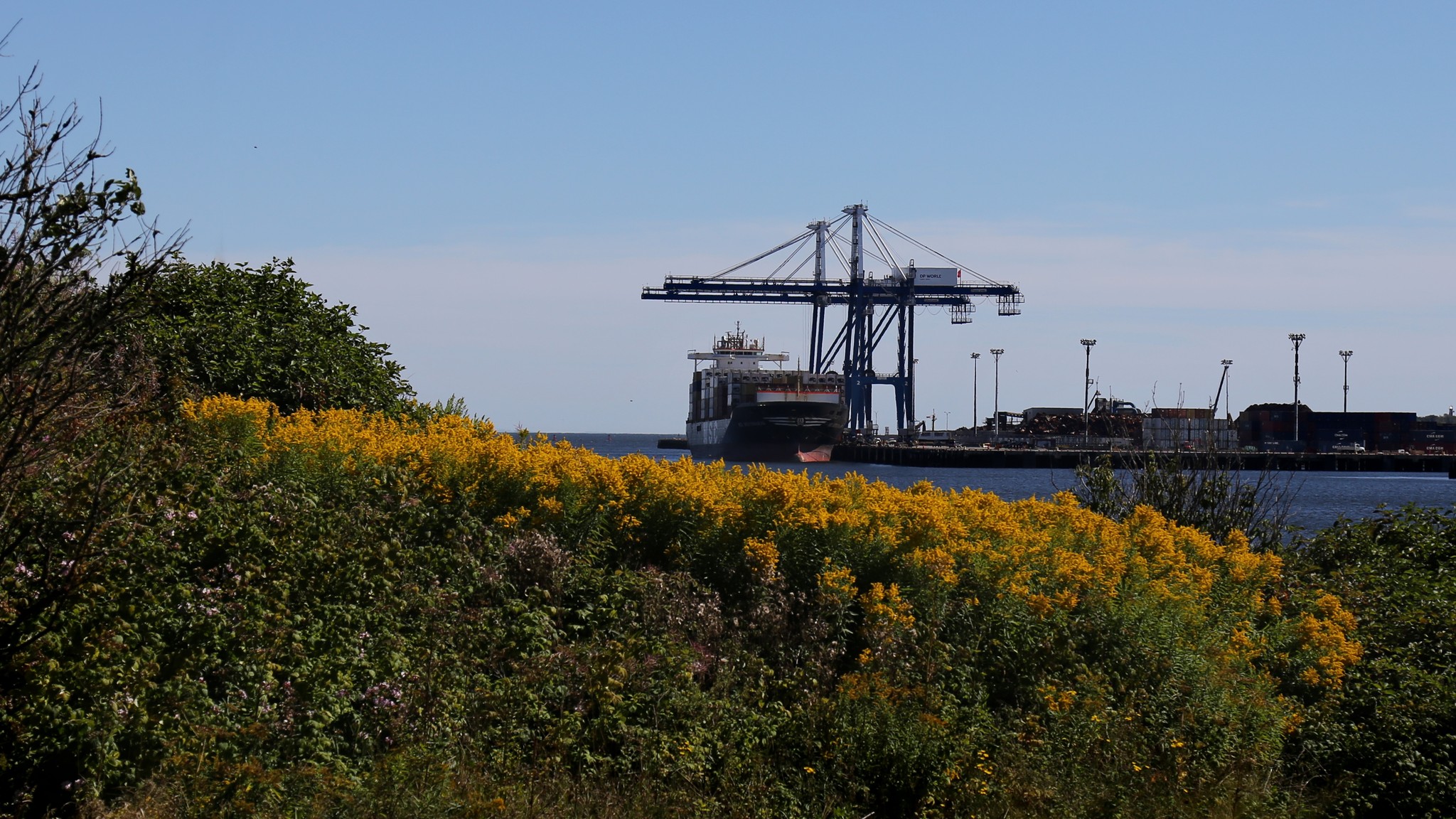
[888,438,896,442]
[874,437,881,444]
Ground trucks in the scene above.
[1333,442,1365,454]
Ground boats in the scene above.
[552,439,557,441]
[792,444,833,463]
[607,438,612,440]
[686,321,846,462]
[657,437,686,448]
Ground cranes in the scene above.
[641,203,1025,448]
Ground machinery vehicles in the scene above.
[1181,438,1202,450]
[1213,365,1230,412]
[1084,389,1101,415]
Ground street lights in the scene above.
[1340,350,1353,412]
[970,352,981,436]
[944,411,951,430]
[1220,358,1232,423]
[913,358,919,432]
[1288,332,1306,439]
[873,410,879,428]
[990,348,1004,441]
[1080,338,1097,450]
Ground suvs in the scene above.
[948,440,962,448]
